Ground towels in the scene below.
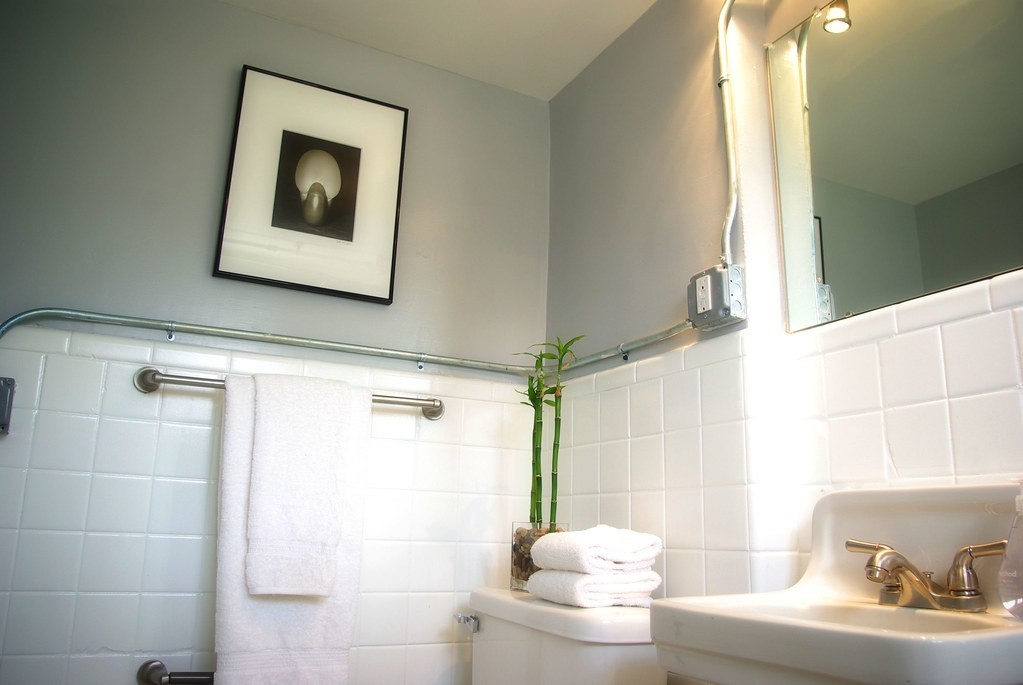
[530,524,663,574]
[213,373,374,685]
[527,569,662,608]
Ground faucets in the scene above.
[844,539,1009,613]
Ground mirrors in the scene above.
[765,0,1023,334]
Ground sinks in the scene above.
[650,597,1022,685]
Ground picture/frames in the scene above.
[813,215,824,285]
[211,64,409,305]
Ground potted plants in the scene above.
[500,335,587,589]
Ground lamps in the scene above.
[815,0,852,35]
